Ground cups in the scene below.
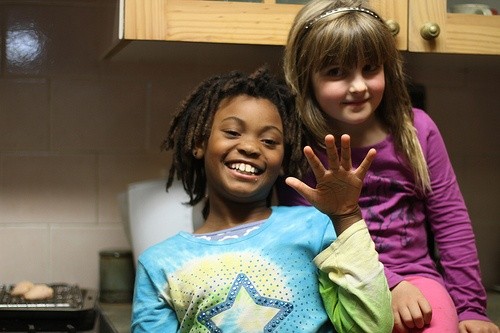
[98,250,134,304]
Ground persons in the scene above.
[128,69,396,333]
[272,0,500,333]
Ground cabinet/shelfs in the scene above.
[95,0,500,80]
